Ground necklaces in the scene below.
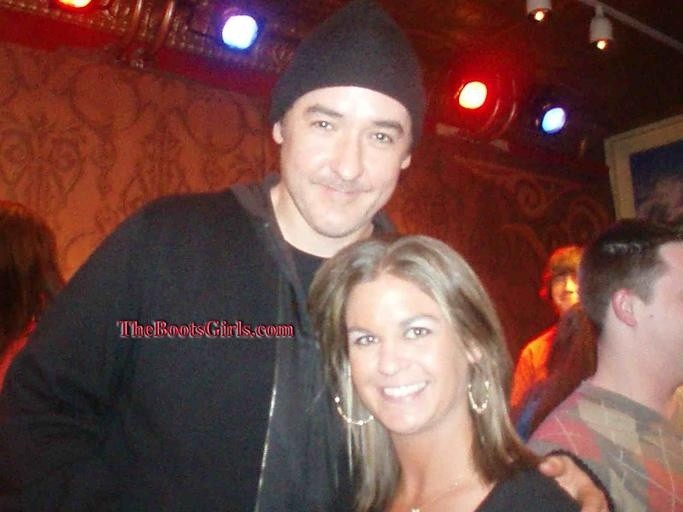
[397,470,476,512]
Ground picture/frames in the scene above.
[605,115,683,220]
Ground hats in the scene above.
[268,2,429,143]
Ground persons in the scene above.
[1,10,619,512]
[507,245,586,413]
[0,198,66,394]
[525,216,683,512]
[510,302,601,443]
[306,233,585,512]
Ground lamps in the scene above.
[535,96,569,137]
[216,0,262,52]
[589,5,613,50]
[525,0,552,22]
